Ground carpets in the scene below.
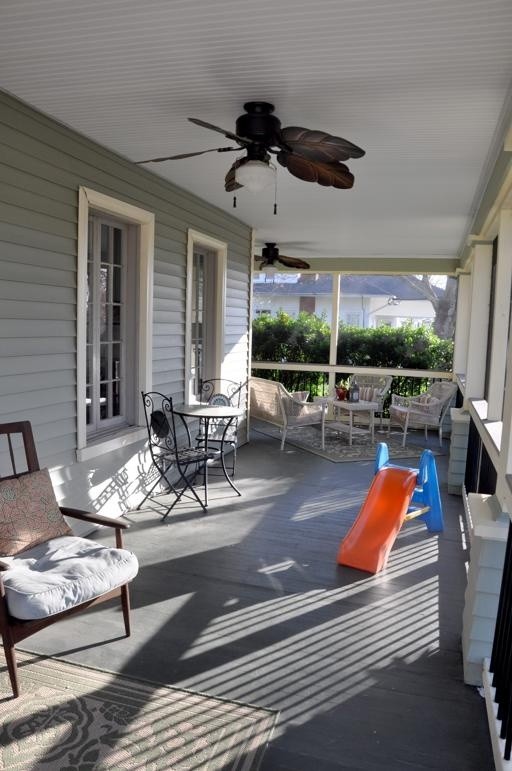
[0,643,281,771]
[251,423,447,464]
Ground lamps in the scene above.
[232,144,278,215]
[137,391,215,522]
[263,259,277,285]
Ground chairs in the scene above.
[193,378,242,486]
[0,420,131,699]
[247,374,459,451]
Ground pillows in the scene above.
[0,467,76,559]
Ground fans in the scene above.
[254,242,310,270]
[132,101,366,193]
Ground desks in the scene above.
[167,404,243,507]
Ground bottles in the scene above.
[349,375,359,402]
